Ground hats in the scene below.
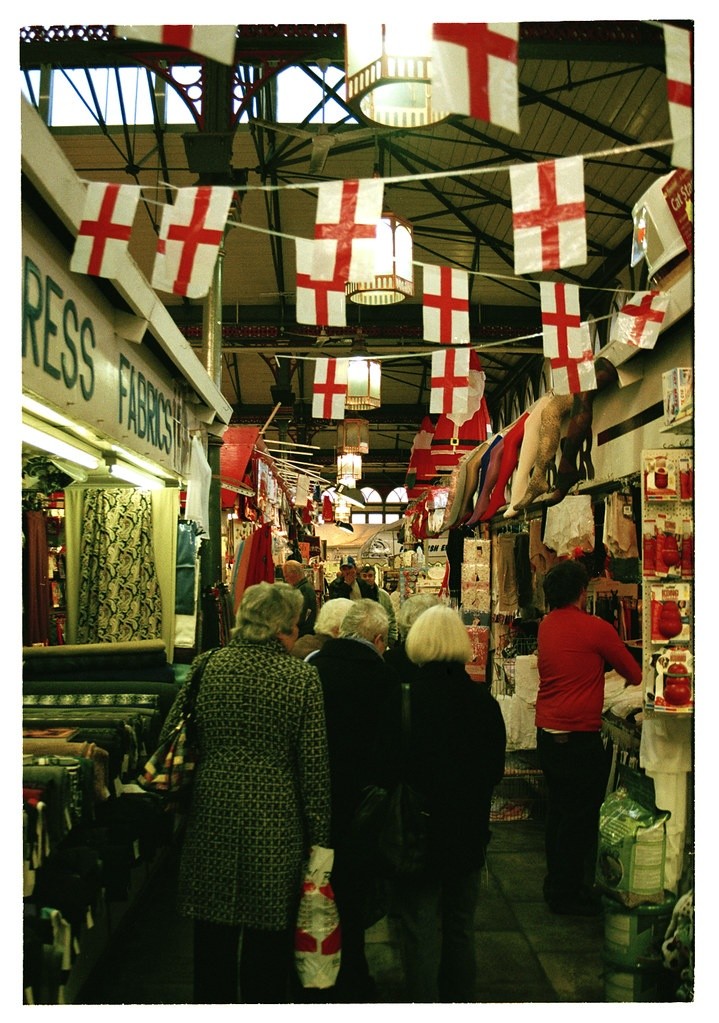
[340,555,355,566]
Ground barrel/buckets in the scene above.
[596,893,677,964]
[598,948,677,1002]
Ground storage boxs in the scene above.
[662,367,692,426]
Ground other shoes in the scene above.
[552,897,604,916]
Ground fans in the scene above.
[249,58,398,175]
[284,325,368,348]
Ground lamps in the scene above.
[336,411,369,455]
[252,448,366,509]
[334,520,353,533]
[343,130,415,306]
[22,411,102,470]
[344,305,381,410]
[105,457,165,492]
[344,24,456,128]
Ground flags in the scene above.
[540,281,583,358]
[509,155,588,274]
[115,24,238,67]
[613,291,669,349]
[151,186,235,299]
[429,349,471,414]
[663,25,694,170]
[429,22,519,134]
[311,358,349,420]
[311,180,384,282]
[295,239,346,327]
[422,266,471,344]
[70,182,141,279]
[550,322,597,394]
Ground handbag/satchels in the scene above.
[136,646,219,799]
[293,845,339,988]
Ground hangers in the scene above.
[604,478,631,502]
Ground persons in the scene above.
[160,557,507,1003]
[531,559,642,916]
[438,358,618,533]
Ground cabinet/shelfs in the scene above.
[489,637,549,824]
